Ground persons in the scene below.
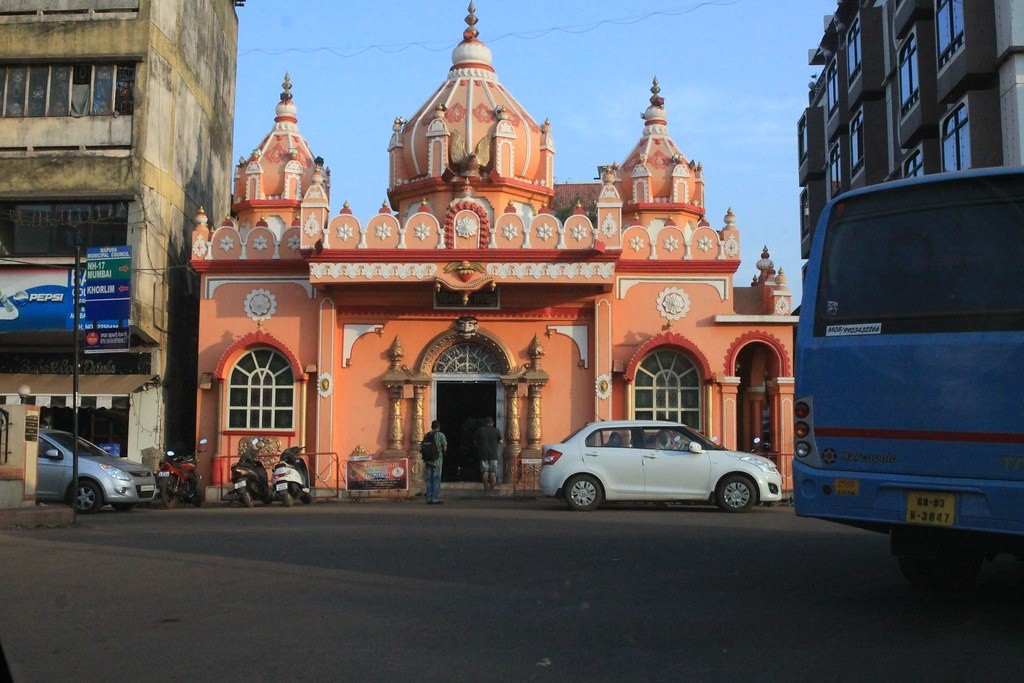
[474,417,503,496]
[421,420,447,504]
[605,432,621,447]
[645,435,658,448]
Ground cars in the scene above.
[35,427,156,515]
[537,419,784,514]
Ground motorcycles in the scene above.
[269,446,313,507]
[229,441,272,508]
[156,438,208,509]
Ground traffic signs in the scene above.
[82,246,133,354]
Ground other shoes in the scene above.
[489,492,497,496]
[481,493,489,497]
[427,498,431,502]
[432,499,439,502]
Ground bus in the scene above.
[790,163,1024,598]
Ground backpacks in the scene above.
[419,431,439,461]
